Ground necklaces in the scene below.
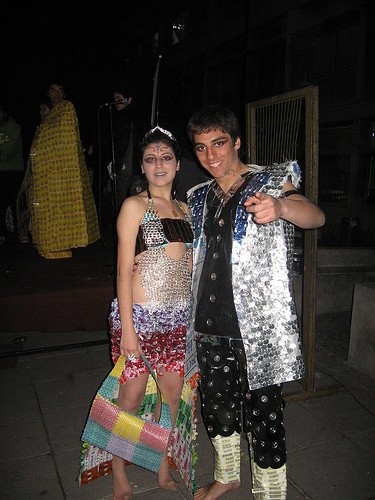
[145,189,178,217]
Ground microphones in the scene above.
[100,99,128,108]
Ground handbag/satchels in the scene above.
[81,351,173,474]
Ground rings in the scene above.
[129,354,135,361]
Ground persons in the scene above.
[133,110,325,500]
[0,80,140,275]
[72,125,199,500]
